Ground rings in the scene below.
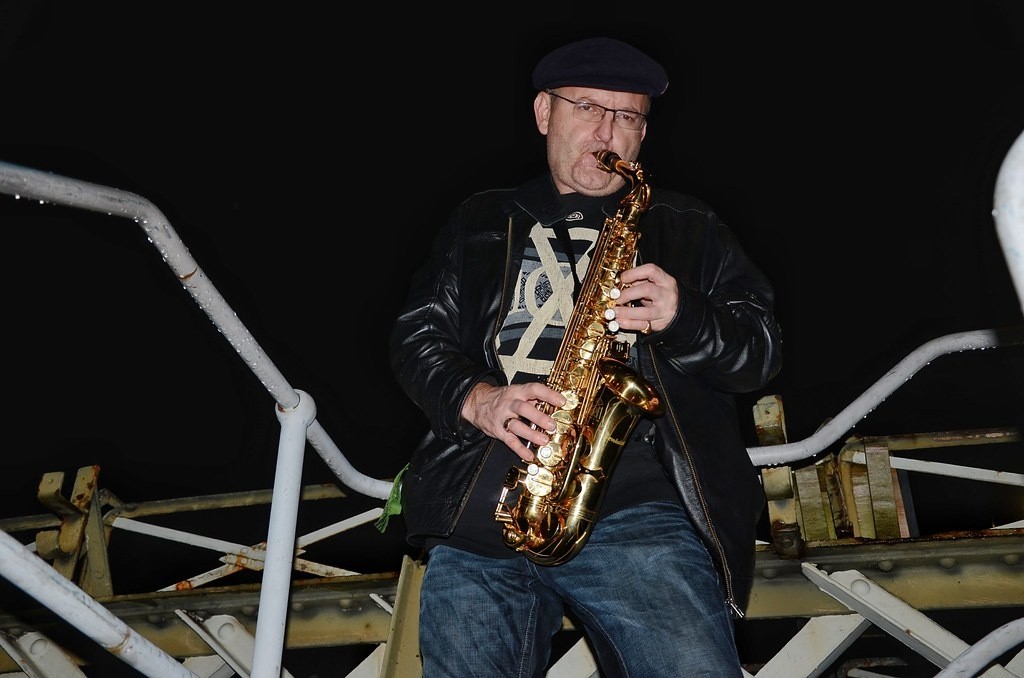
[503,417,519,433]
[641,320,654,335]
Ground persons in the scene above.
[385,36,790,678]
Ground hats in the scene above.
[534,38,669,95]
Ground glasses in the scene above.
[546,91,646,131]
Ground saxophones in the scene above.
[493,148,668,566]
